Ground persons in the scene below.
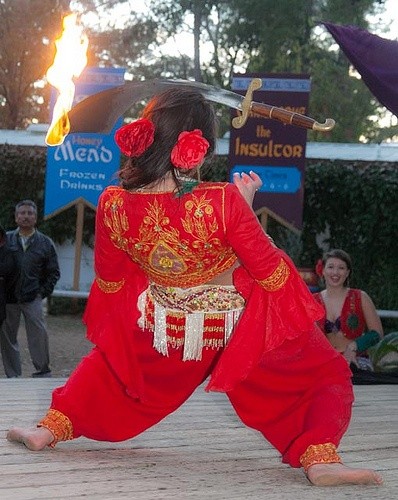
[0,223,20,328]
[0,200,62,379]
[309,250,384,386]
[0,86,384,487]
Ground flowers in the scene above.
[170,127,211,171]
[114,119,157,158]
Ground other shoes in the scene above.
[31,366,51,377]
